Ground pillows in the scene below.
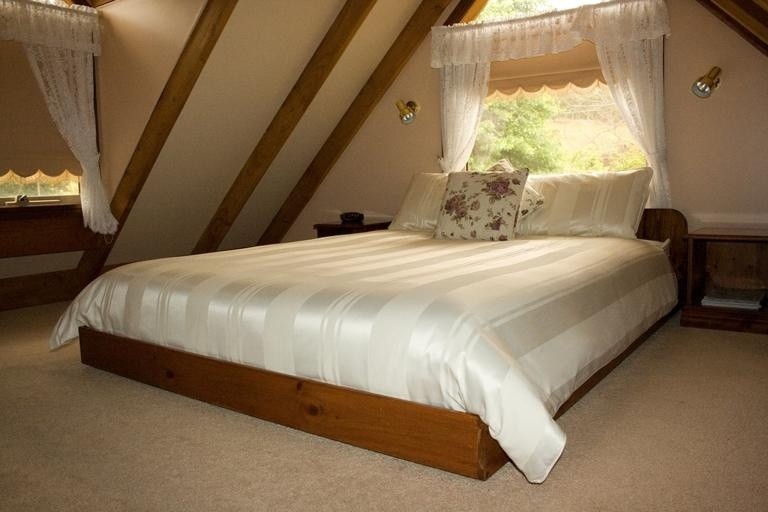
[388,157,655,241]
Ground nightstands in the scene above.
[313,219,391,237]
[679,227,768,334]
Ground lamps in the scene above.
[690,65,722,98]
[395,98,420,125]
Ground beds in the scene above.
[76,207,690,483]
